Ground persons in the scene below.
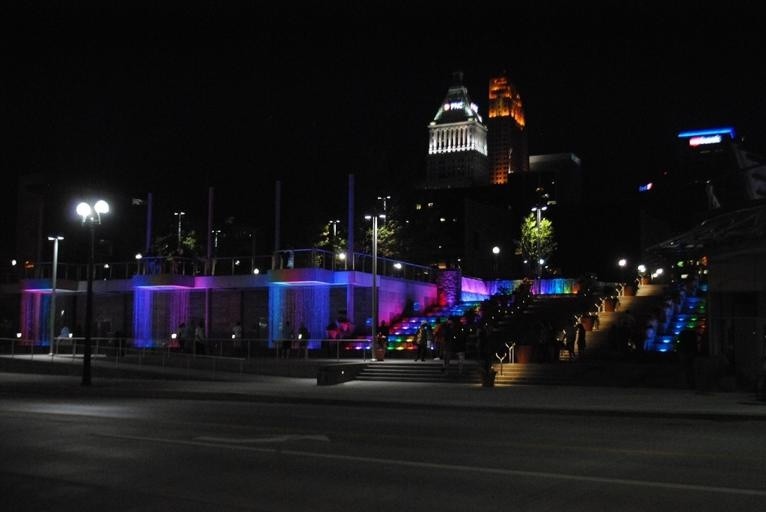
[562,324,577,360]
[231,321,242,351]
[378,320,389,347]
[281,321,294,359]
[112,327,125,357]
[299,322,310,358]
[177,323,189,352]
[195,320,207,355]
[413,314,465,361]
[577,324,587,357]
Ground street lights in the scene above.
[329,219,341,236]
[45,234,67,355]
[491,245,501,279]
[211,229,222,258]
[74,199,113,387]
[377,195,392,227]
[363,213,386,363]
[531,206,548,295]
[174,210,186,241]
